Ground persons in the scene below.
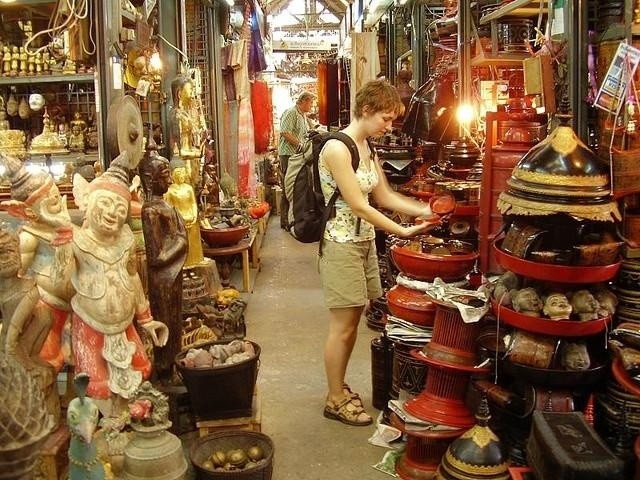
[493,270,618,322]
[0,19,202,480]
[309,79,452,426]
[305,110,319,129]
[278,91,317,232]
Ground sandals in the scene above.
[323,383,372,427]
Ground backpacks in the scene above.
[284,130,376,243]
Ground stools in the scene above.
[196,393,261,438]
[202,229,257,293]
